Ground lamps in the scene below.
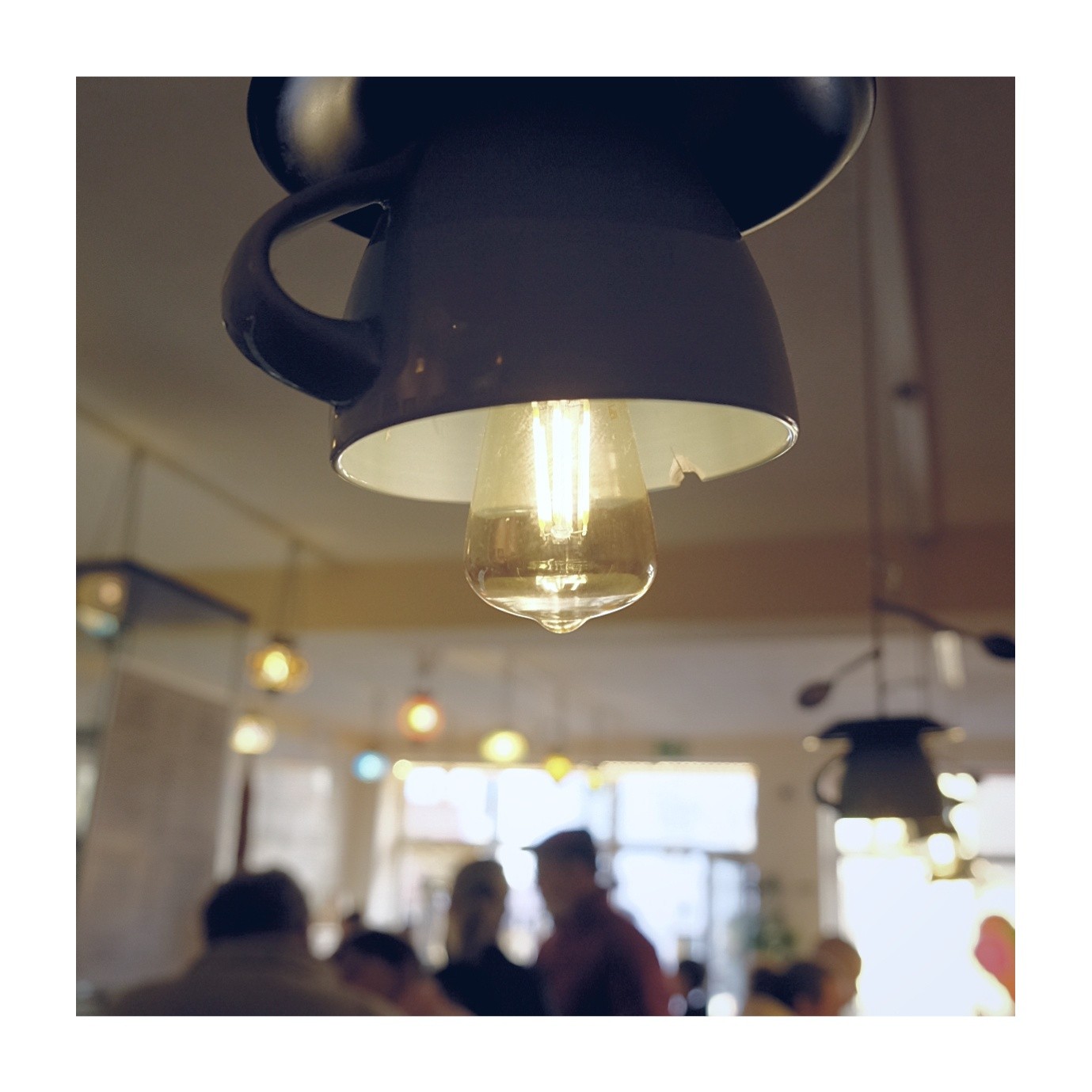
[227,76,885,636]
[809,78,948,849]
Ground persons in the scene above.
[678,952,706,1016]
[435,860,535,1016]
[742,937,862,1016]
[326,911,424,1000]
[521,828,670,1016]
[100,868,400,1016]
